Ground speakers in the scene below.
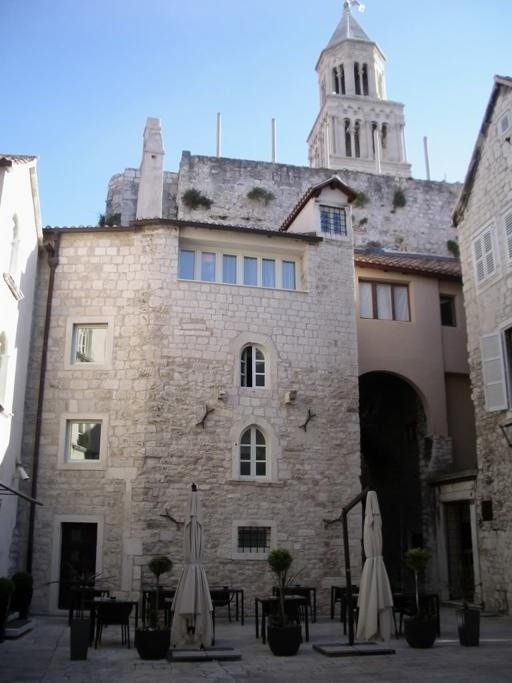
[481,500,493,521]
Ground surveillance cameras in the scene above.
[17,466,30,481]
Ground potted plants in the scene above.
[134,555,174,661]
[40,564,119,661]
[265,548,303,656]
[0,571,33,644]
[402,546,438,649]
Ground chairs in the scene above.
[93,600,131,650]
[141,590,176,629]
[68,589,110,626]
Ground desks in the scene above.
[80,600,139,648]
[254,594,310,644]
[272,586,317,624]
[209,587,245,626]
[330,584,406,641]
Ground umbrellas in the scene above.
[341,485,394,643]
[168,483,215,651]
